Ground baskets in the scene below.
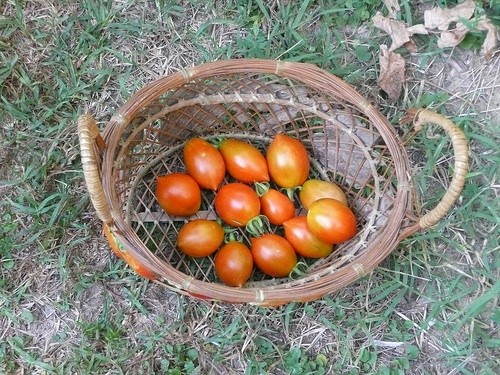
[78,58,468,308]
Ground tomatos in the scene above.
[153,132,356,286]
